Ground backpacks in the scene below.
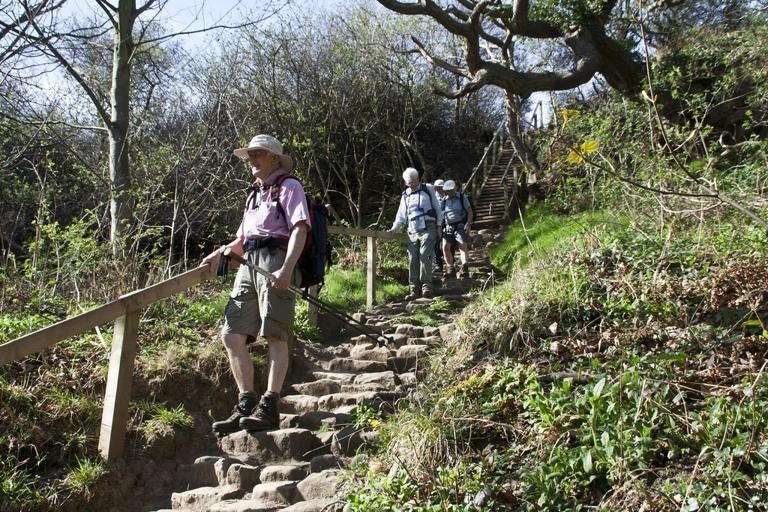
[459,192,476,222]
[269,174,328,288]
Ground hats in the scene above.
[434,179,455,190]
[233,134,294,169]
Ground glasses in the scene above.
[405,182,413,186]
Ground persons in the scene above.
[442,178,474,281]
[200,135,311,432]
[431,179,457,273]
[386,168,445,301]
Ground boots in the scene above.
[212,391,281,432]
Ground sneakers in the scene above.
[433,266,469,277]
[405,284,433,300]
